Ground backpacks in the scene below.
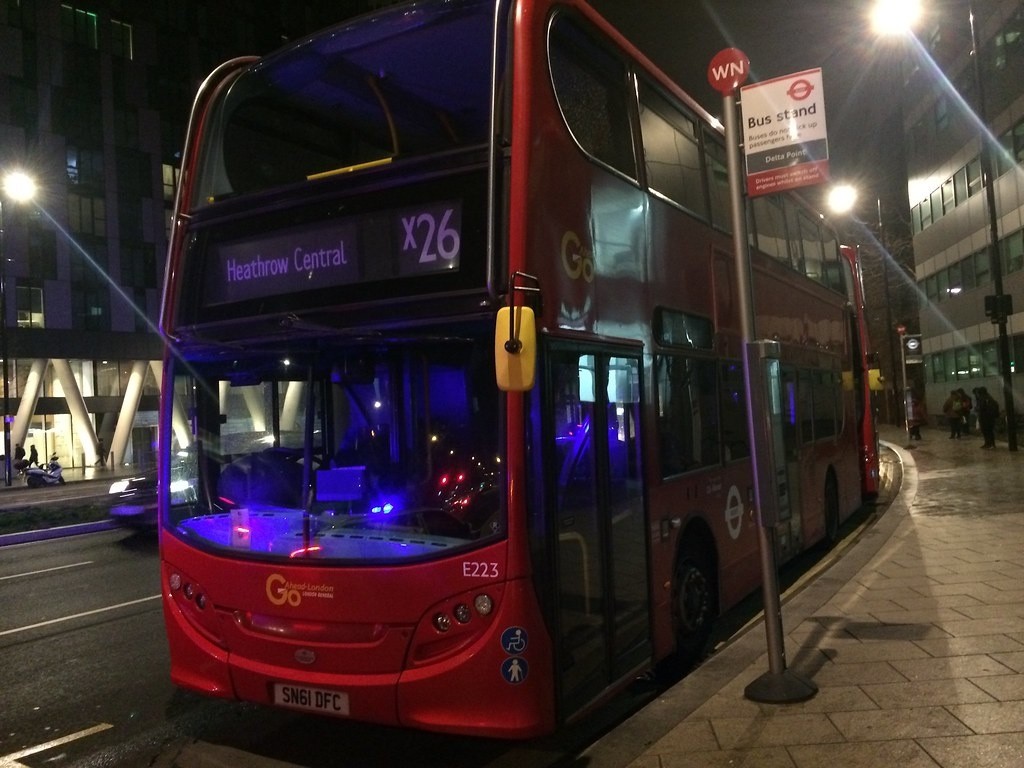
[950,396,962,412]
[963,398,972,413]
[979,392,1000,419]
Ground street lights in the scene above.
[0,161,47,485]
[837,0,1016,449]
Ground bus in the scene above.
[158,0,879,741]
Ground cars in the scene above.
[110,430,322,532]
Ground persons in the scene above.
[910,387,998,450]
[95,438,106,467]
[15,442,40,472]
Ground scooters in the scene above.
[23,452,67,488]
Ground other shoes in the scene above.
[948,436,955,440]
[915,434,921,441]
[956,435,961,439]
[980,444,996,451]
